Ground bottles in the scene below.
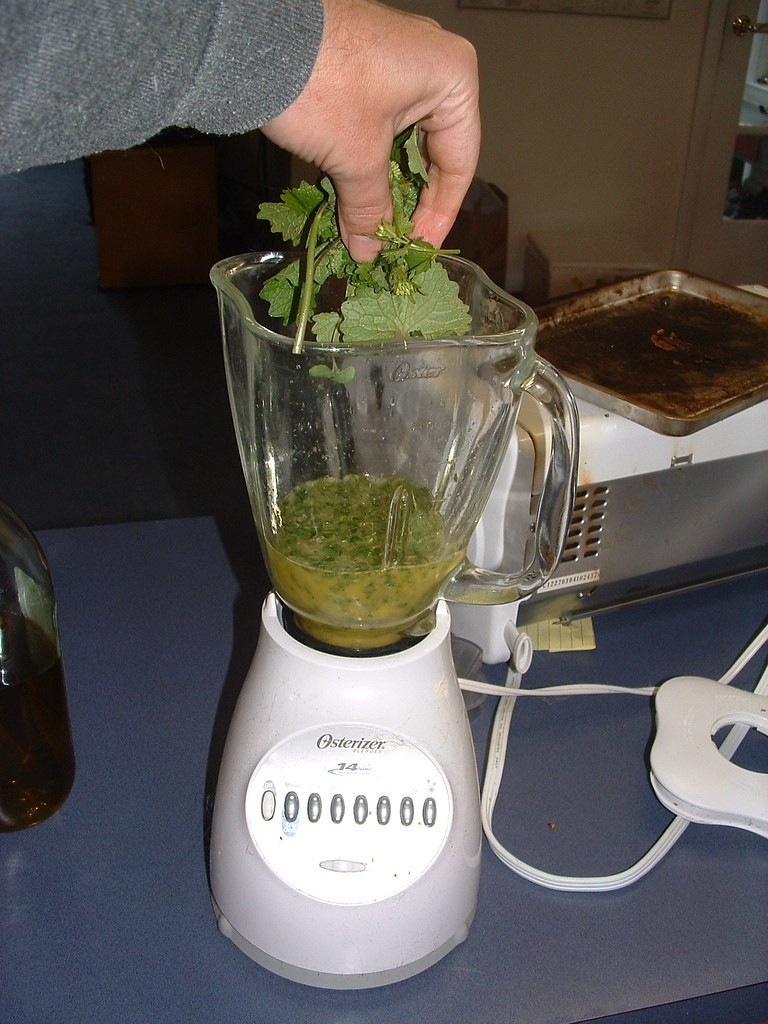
[0,506,78,834]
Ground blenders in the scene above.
[208,240,579,994]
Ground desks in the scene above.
[0,509,768,1024]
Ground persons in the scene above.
[0,0,485,261]
